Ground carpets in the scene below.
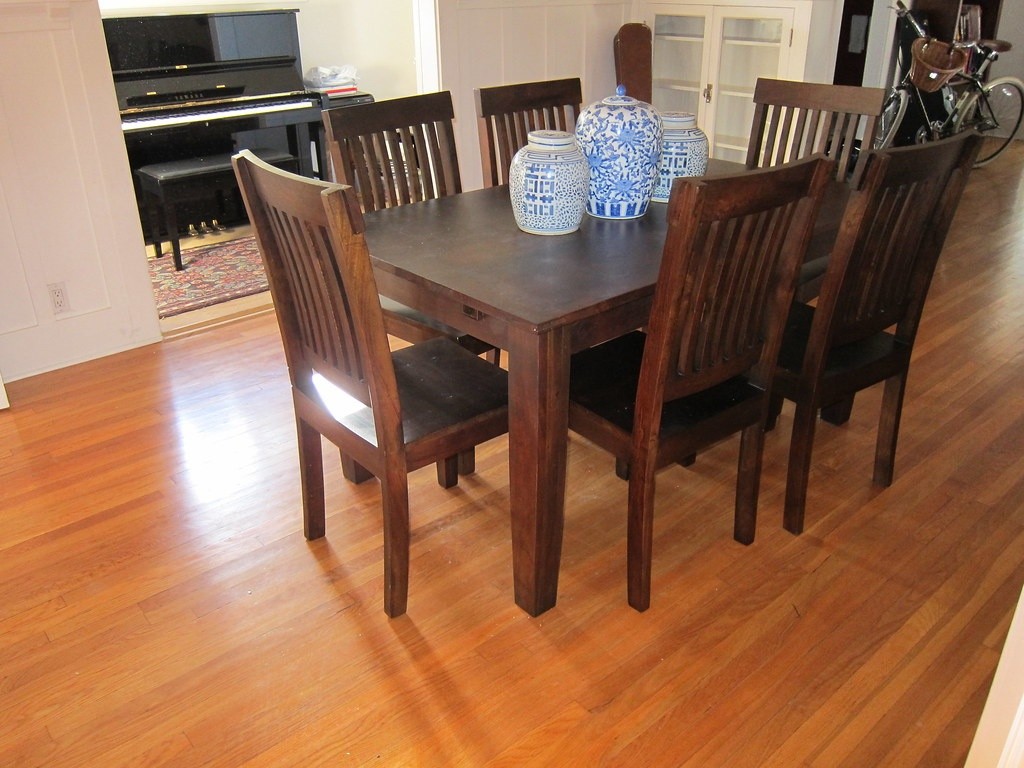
[147,234,269,321]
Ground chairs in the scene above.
[319,89,501,478]
[473,77,584,188]
[744,76,888,193]
[226,148,510,618]
[564,152,833,615]
[758,128,987,537]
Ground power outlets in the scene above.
[47,281,70,315]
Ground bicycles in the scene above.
[873,0,1024,169]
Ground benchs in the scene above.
[138,146,300,271]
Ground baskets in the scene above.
[910,38,967,92]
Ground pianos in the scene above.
[100,6,333,273]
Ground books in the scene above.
[955,4,981,41]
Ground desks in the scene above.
[340,157,850,619]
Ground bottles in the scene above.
[574,84,664,221]
[508,129,591,236]
[651,110,710,204]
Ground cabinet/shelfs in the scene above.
[892,0,1005,147]
[630,0,836,164]
